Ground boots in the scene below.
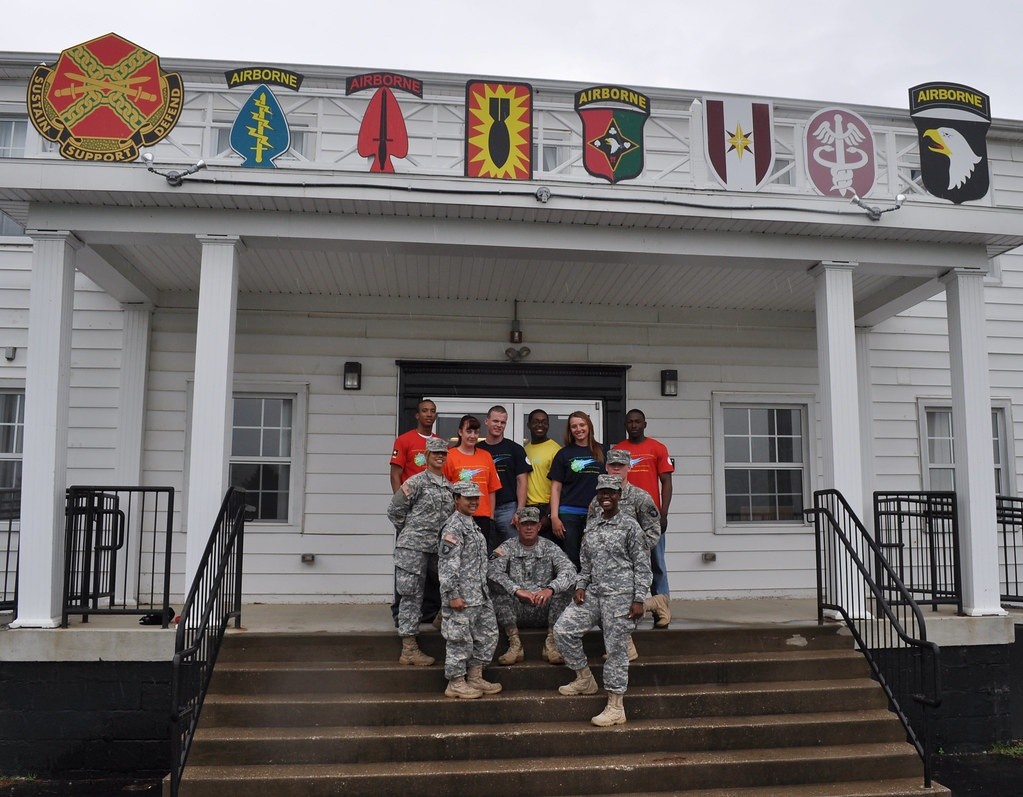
[498,634,523,665]
[645,594,671,626]
[541,627,562,663]
[601,635,637,660]
[445,679,482,699]
[591,692,626,726]
[432,607,442,632]
[399,636,434,666]
[559,665,598,695]
[466,665,502,693]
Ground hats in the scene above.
[452,481,484,497]
[426,437,448,453]
[517,507,539,522]
[595,474,622,490]
[606,450,630,465]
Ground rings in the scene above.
[540,596,543,599]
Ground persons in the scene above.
[387,437,455,667]
[487,506,578,664]
[523,409,563,539]
[389,399,442,629]
[475,405,535,539]
[586,448,671,659]
[436,480,502,698]
[611,409,674,596]
[441,415,502,545]
[547,411,608,572]
[553,474,653,726]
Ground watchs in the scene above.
[546,513,551,518]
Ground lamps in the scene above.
[505,347,530,362]
[141,152,207,185]
[511,300,522,343]
[849,193,907,221]
[661,370,678,396]
[342,361,362,390]
